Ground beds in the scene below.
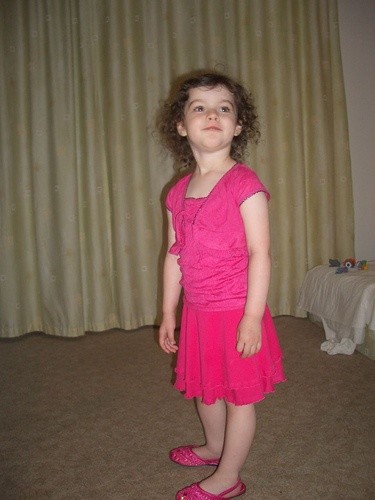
[298,260,375,361]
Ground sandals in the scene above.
[175,479,246,500]
[169,445,221,466]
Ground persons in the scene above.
[157,69,286,500]
